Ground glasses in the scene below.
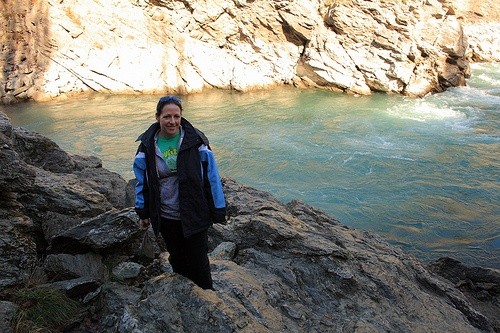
[156,96,181,111]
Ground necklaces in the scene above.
[130,95,226,293]
